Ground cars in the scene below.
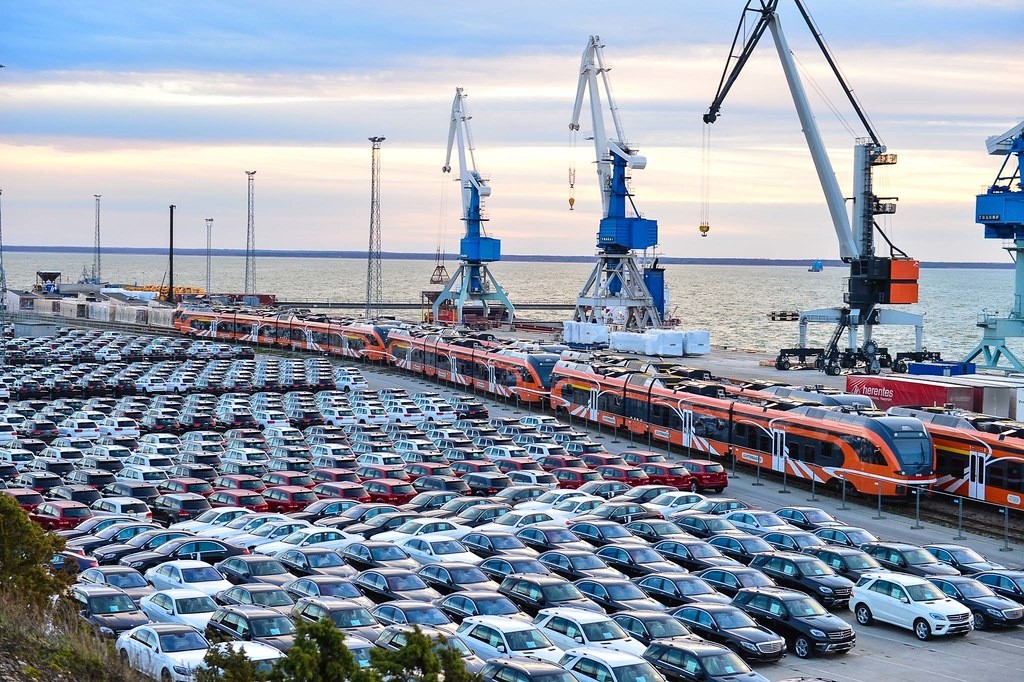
[1,290,1024,682]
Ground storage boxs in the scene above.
[845,361,1024,423]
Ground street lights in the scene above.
[203,217,214,300]
[362,136,388,320]
[244,169,258,297]
[91,193,102,283]
[0,189,6,292]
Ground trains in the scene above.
[171,307,937,506]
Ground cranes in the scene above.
[431,86,514,329]
[700,0,927,362]
[567,35,664,331]
[961,120,1024,373]
